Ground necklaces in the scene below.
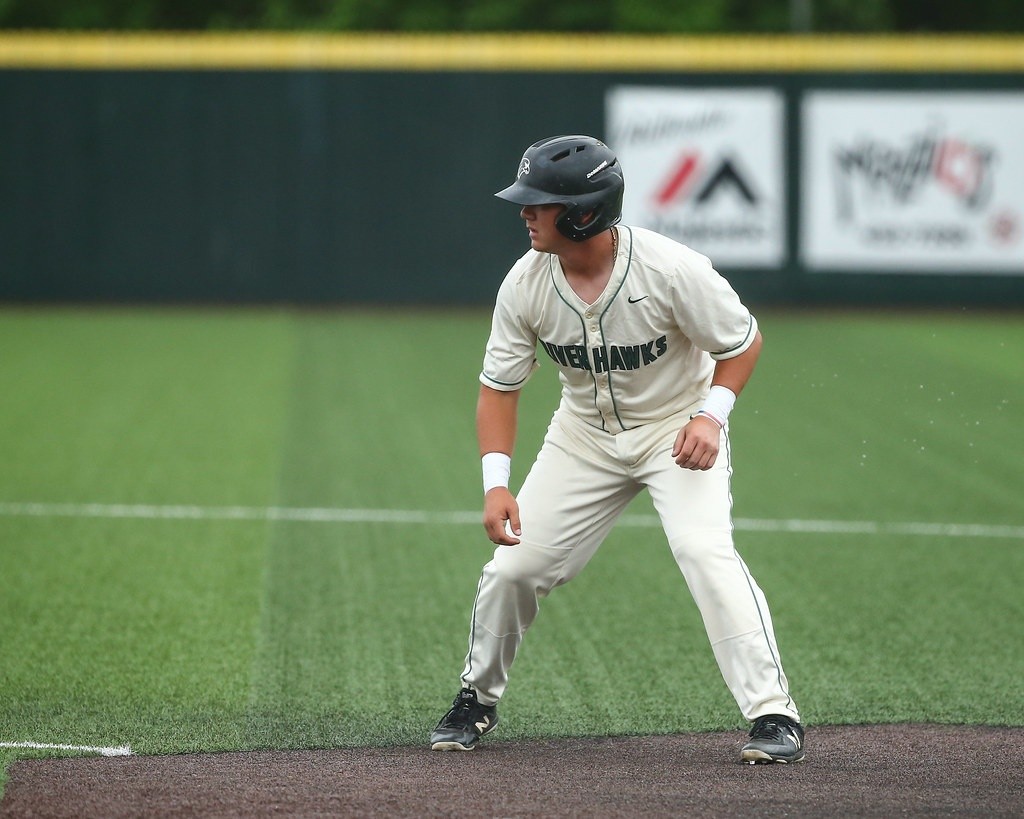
[609,228,616,265]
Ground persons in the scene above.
[429,134,806,762]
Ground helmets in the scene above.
[494,135,624,242]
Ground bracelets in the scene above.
[693,385,737,428]
[482,451,511,495]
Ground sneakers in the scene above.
[740,715,805,765]
[431,688,498,750]
